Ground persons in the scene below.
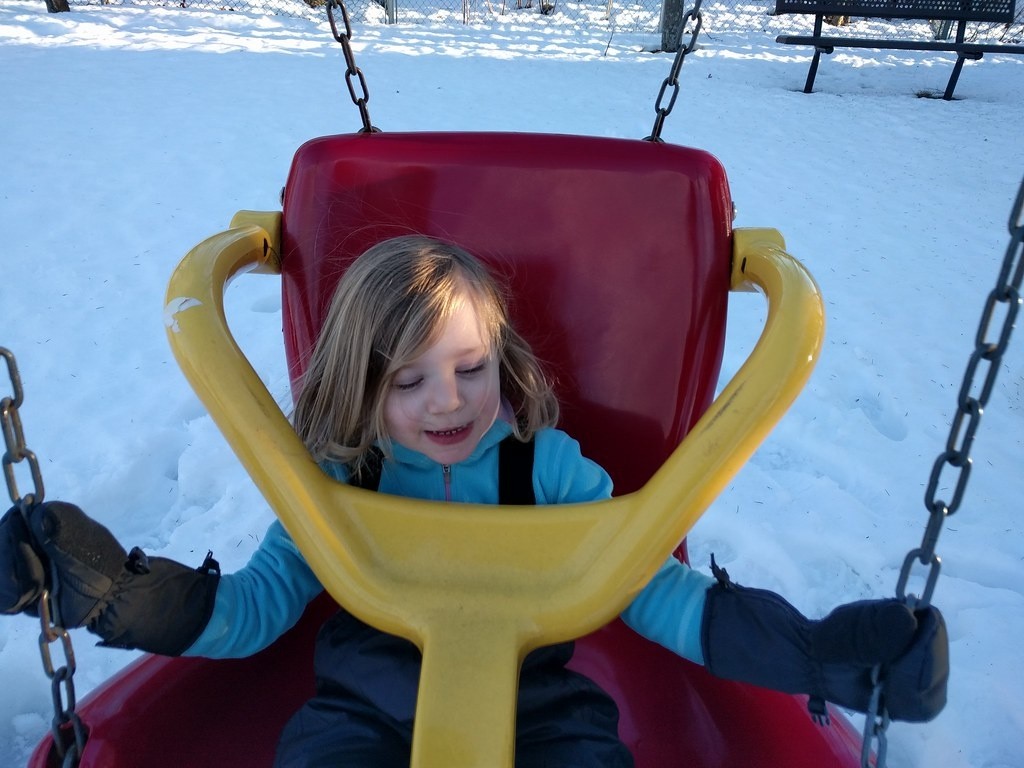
[0,233,953,767]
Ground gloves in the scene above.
[0,502,221,656]
[700,553,950,723]
